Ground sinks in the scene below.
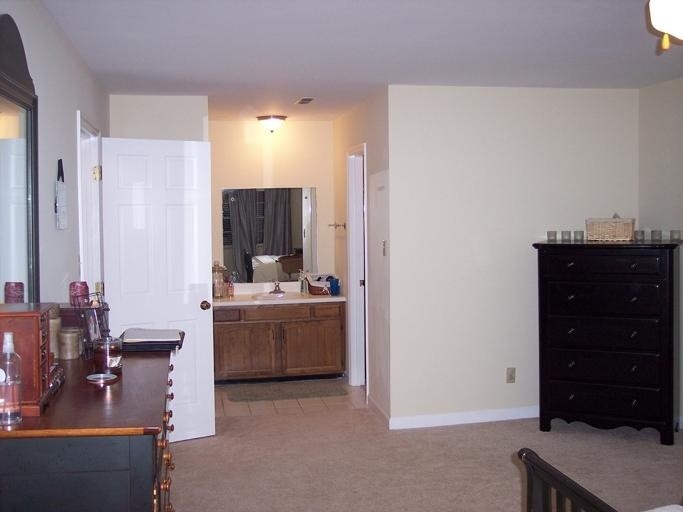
[252,292,304,300]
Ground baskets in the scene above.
[306,273,340,295]
[586,218,635,241]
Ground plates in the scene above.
[87,373,119,381]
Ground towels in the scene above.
[123,328,181,342]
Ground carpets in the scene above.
[225,379,346,401]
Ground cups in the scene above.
[669,230,681,244]
[573,231,584,242]
[93,338,123,368]
[546,231,557,243]
[633,230,644,242]
[651,230,662,242]
[561,231,571,243]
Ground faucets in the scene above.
[269,281,285,294]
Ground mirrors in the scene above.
[0,12,40,302]
[221,186,316,284]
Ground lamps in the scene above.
[257,115,285,133]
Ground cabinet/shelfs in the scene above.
[1,304,50,416]
[532,240,683,446]
[1,349,176,512]
[213,301,346,380]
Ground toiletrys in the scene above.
[0,331,21,425]
[226,279,235,296]
